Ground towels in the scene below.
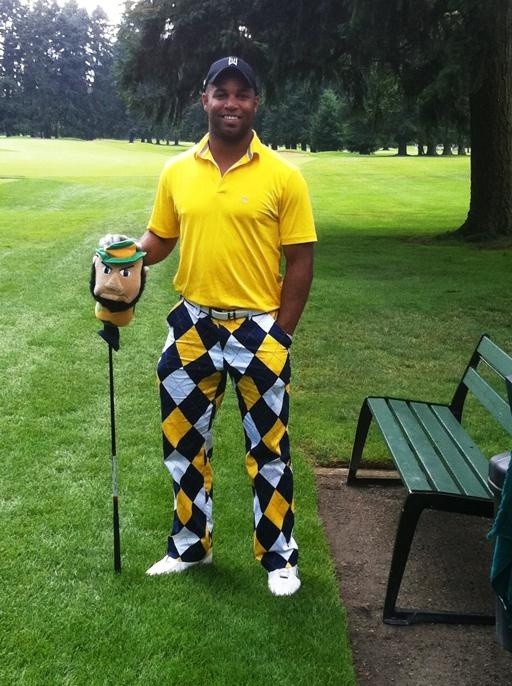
[486,449,512,612]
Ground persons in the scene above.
[90,240,149,353]
[98,55,318,600]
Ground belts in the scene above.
[182,298,267,320]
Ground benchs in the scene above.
[346,332,512,623]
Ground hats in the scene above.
[203,56,257,94]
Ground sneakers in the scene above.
[267,564,301,597]
[146,548,213,575]
